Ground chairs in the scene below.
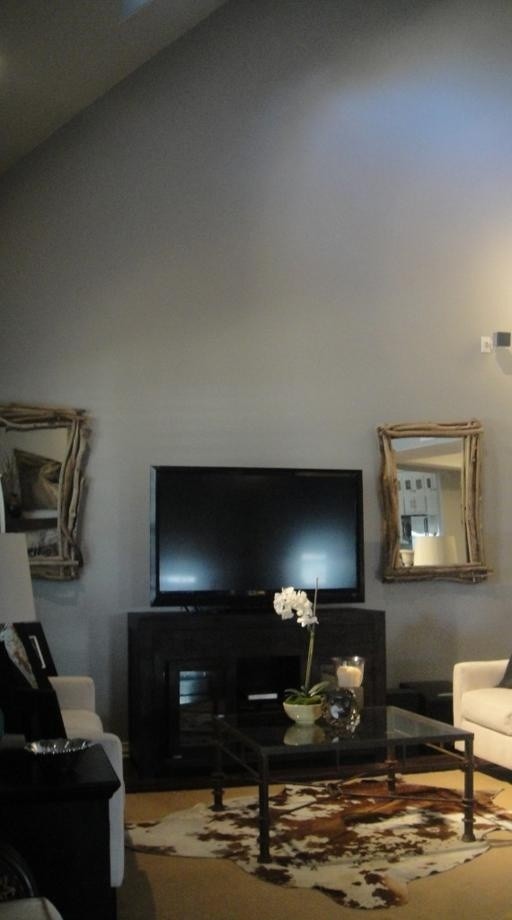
[453,659,510,775]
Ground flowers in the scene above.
[271,586,319,629]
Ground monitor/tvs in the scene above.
[149,463,365,611]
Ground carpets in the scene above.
[126,776,510,911]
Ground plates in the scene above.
[23,737,98,764]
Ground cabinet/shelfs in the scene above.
[126,608,386,790]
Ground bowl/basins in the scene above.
[284,726,325,746]
[281,702,323,726]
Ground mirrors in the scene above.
[377,419,493,586]
[2,403,95,581]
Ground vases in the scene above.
[283,695,322,726]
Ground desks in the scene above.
[208,705,476,864]
